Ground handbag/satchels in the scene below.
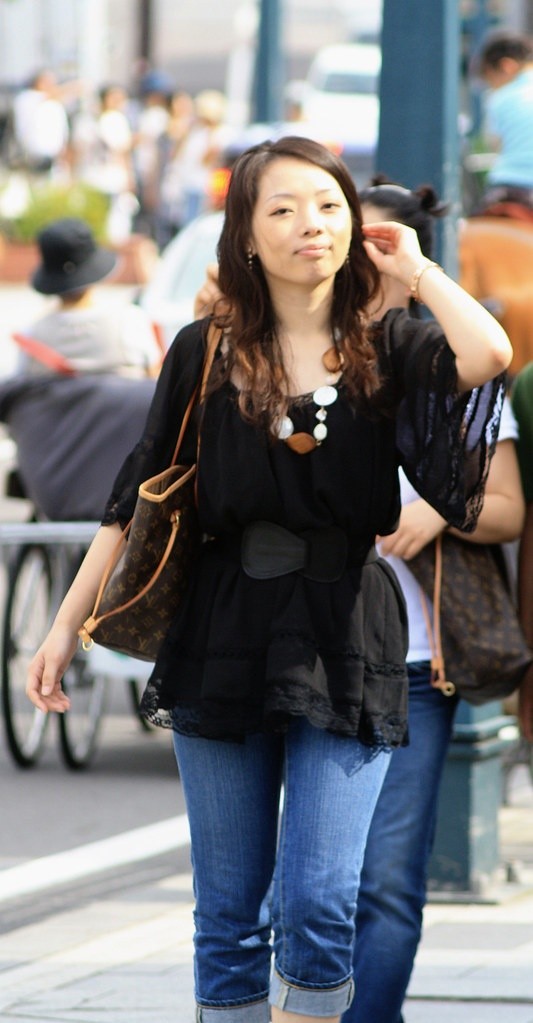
[79,303,233,662]
[403,524,533,707]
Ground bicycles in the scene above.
[1,470,160,770]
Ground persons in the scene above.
[334,31,533,1023]
[27,136,513,1023]
[0,71,317,384]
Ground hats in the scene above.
[33,220,116,295]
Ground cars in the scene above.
[292,46,383,167]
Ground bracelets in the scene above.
[410,262,442,304]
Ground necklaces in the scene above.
[262,329,345,455]
